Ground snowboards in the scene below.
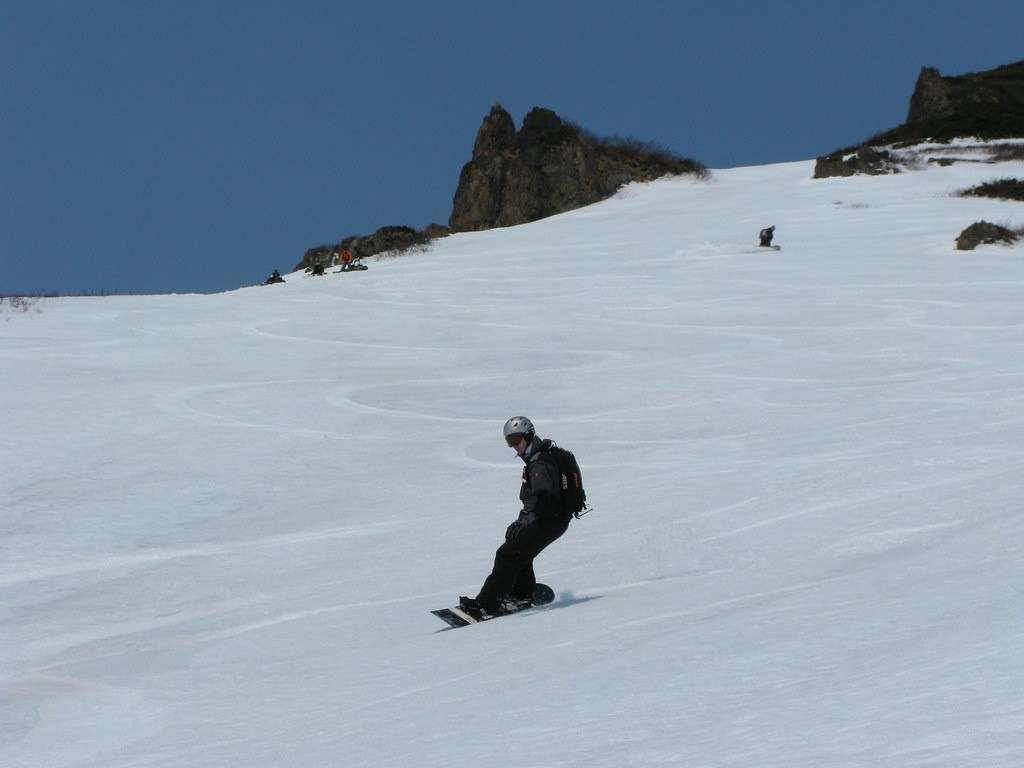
[430,583,555,629]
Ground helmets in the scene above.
[503,416,535,442]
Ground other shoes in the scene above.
[501,595,531,612]
[460,597,496,616]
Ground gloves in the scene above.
[505,509,537,543]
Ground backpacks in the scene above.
[548,444,593,520]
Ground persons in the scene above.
[458,415,573,618]
[266,249,363,284]
[760,226,776,247]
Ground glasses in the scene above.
[505,433,524,447]
